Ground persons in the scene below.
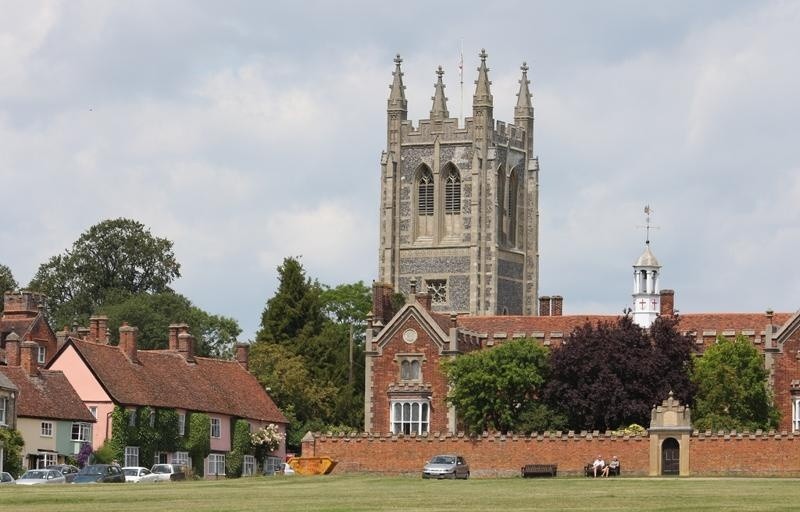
[589,454,605,478]
[601,455,619,477]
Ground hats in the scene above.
[598,455,602,457]
[612,456,617,458]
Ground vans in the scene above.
[150,464,185,483]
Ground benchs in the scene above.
[521,464,557,478]
[584,463,620,476]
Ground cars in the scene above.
[0,472,16,485]
[72,464,125,484]
[122,467,159,484]
[45,465,80,483]
[15,469,66,486]
[422,455,469,480]
